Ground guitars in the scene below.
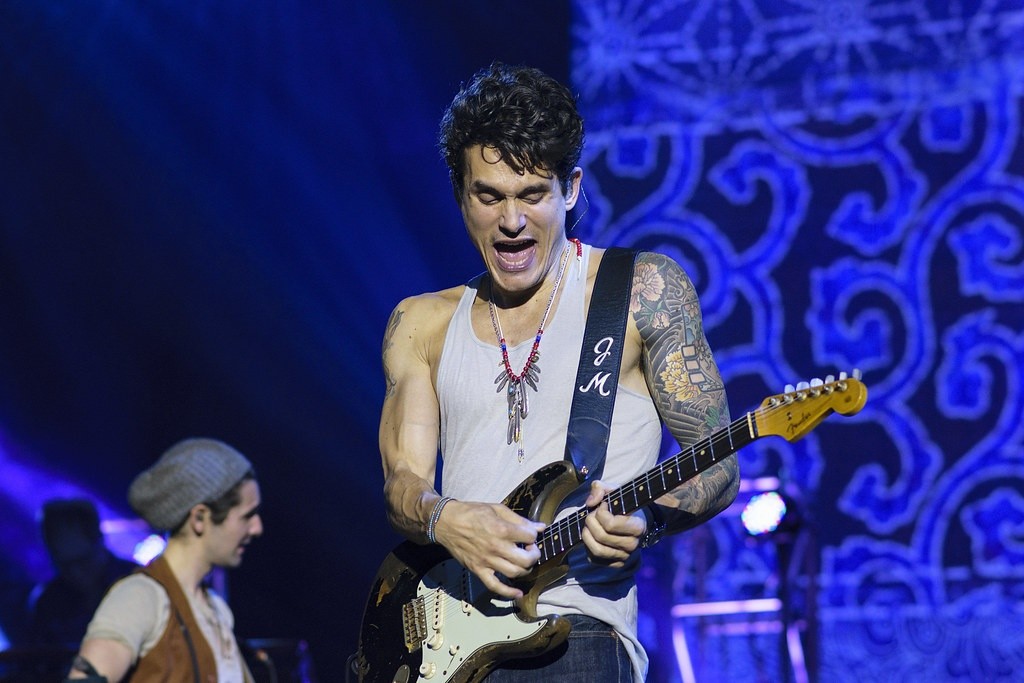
[357,368,869,683]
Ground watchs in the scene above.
[641,502,666,550]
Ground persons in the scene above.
[379,70,740,683]
[14,502,142,683]
[67,439,263,683]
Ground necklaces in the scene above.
[490,239,582,445]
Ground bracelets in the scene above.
[428,496,456,543]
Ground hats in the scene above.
[127,438,254,536]
